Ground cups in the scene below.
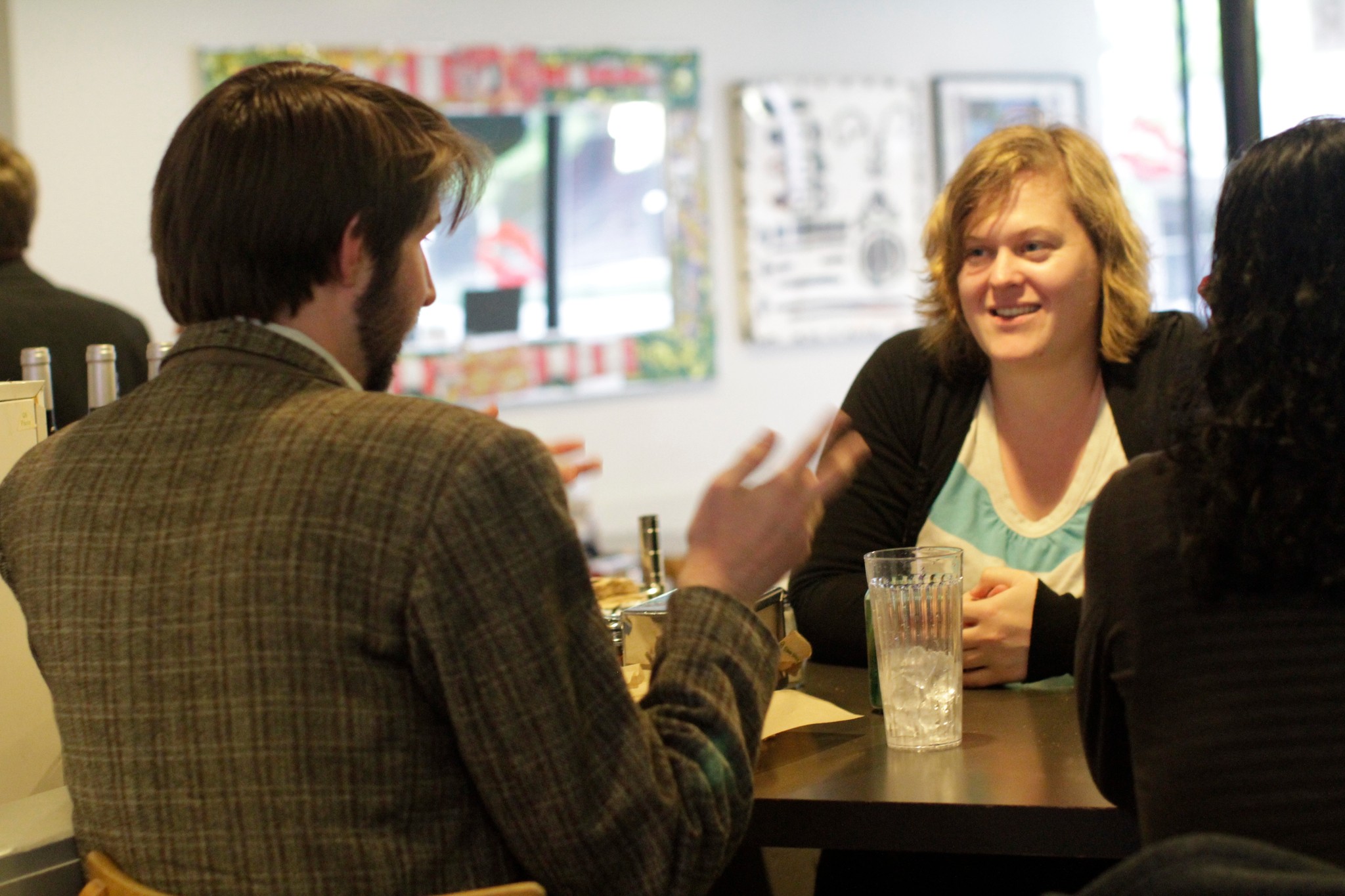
[863,546,964,752]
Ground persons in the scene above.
[1073,122,1345,857]
[0,59,869,896]
[787,124,1207,896]
[0,139,150,431]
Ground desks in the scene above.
[734,656,1163,872]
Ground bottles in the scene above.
[783,597,805,689]
